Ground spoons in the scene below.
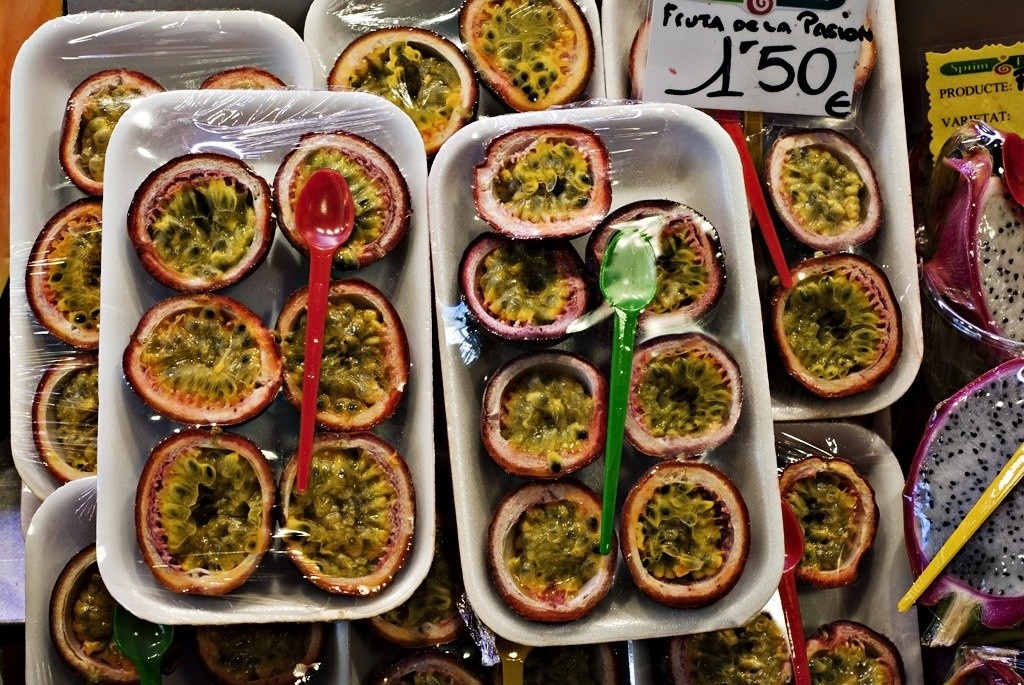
[295,168,355,491]
[599,229,657,556]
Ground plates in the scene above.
[630,417,927,685]
[601,0,926,421]
[302,0,607,117]
[94,90,435,627]
[15,475,398,685]
[429,104,788,646]
[10,11,315,517]
